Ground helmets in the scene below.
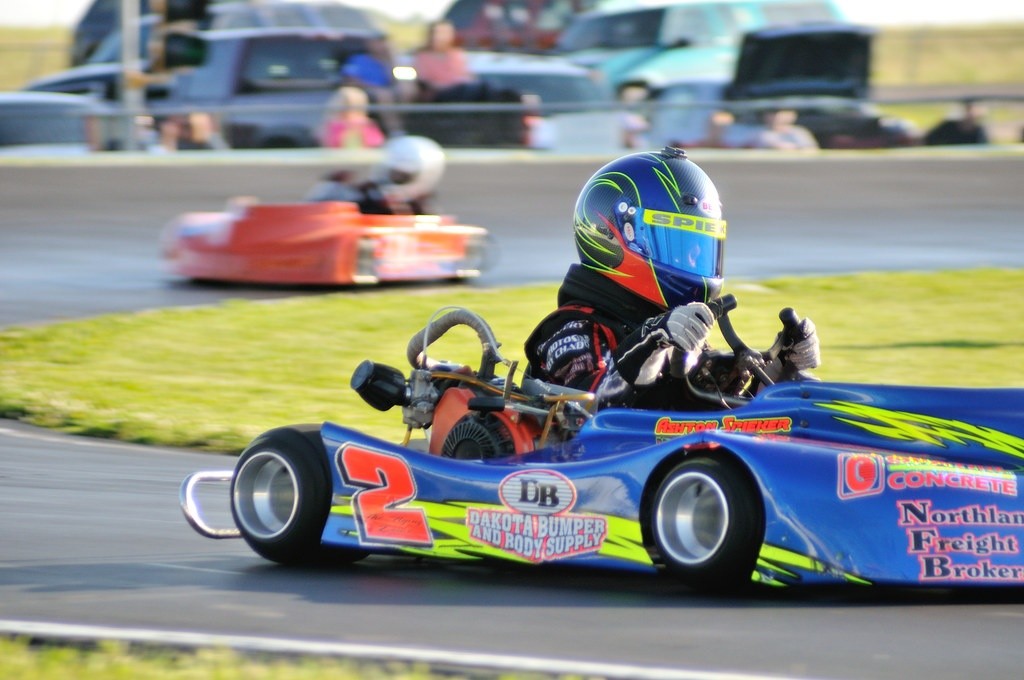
[375,134,445,198]
[572,150,729,306]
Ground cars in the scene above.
[1,1,926,152]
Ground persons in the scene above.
[121,19,201,90]
[330,33,406,134]
[415,20,466,89]
[305,134,445,216]
[160,112,229,151]
[316,88,385,147]
[524,147,822,413]
[613,71,993,150]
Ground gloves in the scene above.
[640,300,716,354]
[769,317,821,371]
